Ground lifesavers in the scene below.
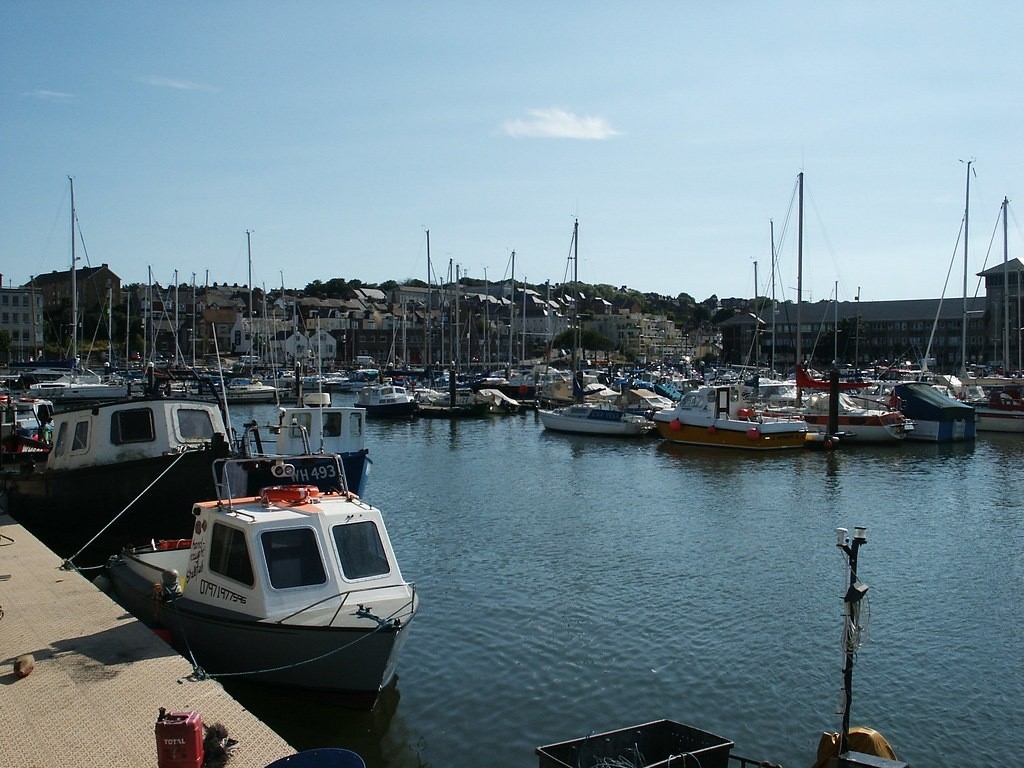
[0,395,12,403]
[261,485,320,501]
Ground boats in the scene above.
[20,177,1024,450]
[40,399,233,543]
[0,394,55,459]
[247,316,374,502]
[107,417,421,713]
